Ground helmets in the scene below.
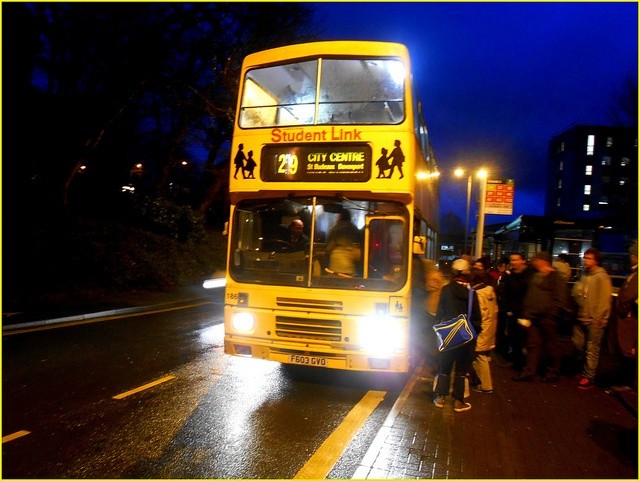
[452,259,470,275]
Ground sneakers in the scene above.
[433,394,445,408]
[454,400,471,412]
[578,377,593,388]
[511,375,534,382]
[471,385,494,394]
[541,371,560,382]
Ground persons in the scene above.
[572,247,612,386]
[474,257,490,270]
[611,240,638,391]
[490,259,506,279]
[325,208,361,277]
[433,259,482,412]
[503,252,531,371]
[551,253,571,281]
[420,258,448,317]
[398,242,427,377]
[511,251,567,383]
[470,267,499,393]
[280,218,310,250]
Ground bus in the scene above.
[224,40,442,375]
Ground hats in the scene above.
[628,240,638,256]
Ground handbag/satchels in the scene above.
[433,313,477,352]
[434,373,470,399]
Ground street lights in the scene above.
[454,167,488,254]
